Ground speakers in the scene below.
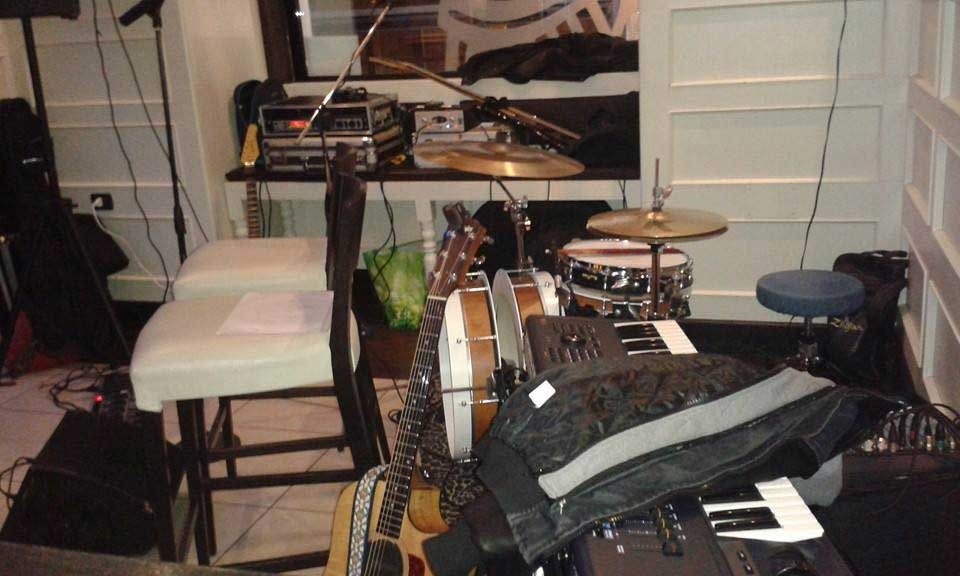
[0,410,185,557]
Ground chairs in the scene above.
[122,169,382,576]
[167,139,363,489]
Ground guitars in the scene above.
[326,204,496,576]
[240,123,262,237]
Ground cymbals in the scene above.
[586,209,727,244]
[411,140,585,177]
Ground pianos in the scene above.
[522,313,857,576]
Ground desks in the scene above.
[222,161,643,281]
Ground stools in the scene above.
[755,270,865,381]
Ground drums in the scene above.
[556,239,693,320]
[492,270,565,385]
[437,270,501,466]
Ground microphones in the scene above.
[119,0,164,26]
